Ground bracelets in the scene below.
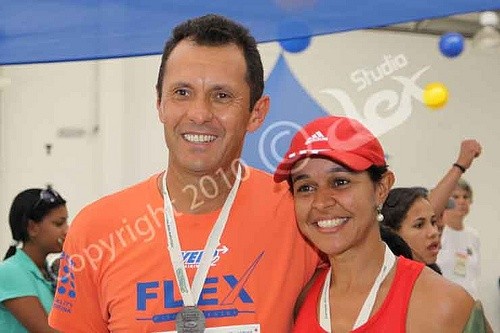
[452,162,465,173]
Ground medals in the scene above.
[175,307,208,332]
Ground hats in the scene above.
[273,116,386,183]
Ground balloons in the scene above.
[422,81,449,108]
[474,26,500,58]
[276,18,312,53]
[439,31,463,57]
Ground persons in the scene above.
[0,12,494,333]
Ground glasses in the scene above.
[32,185,60,213]
